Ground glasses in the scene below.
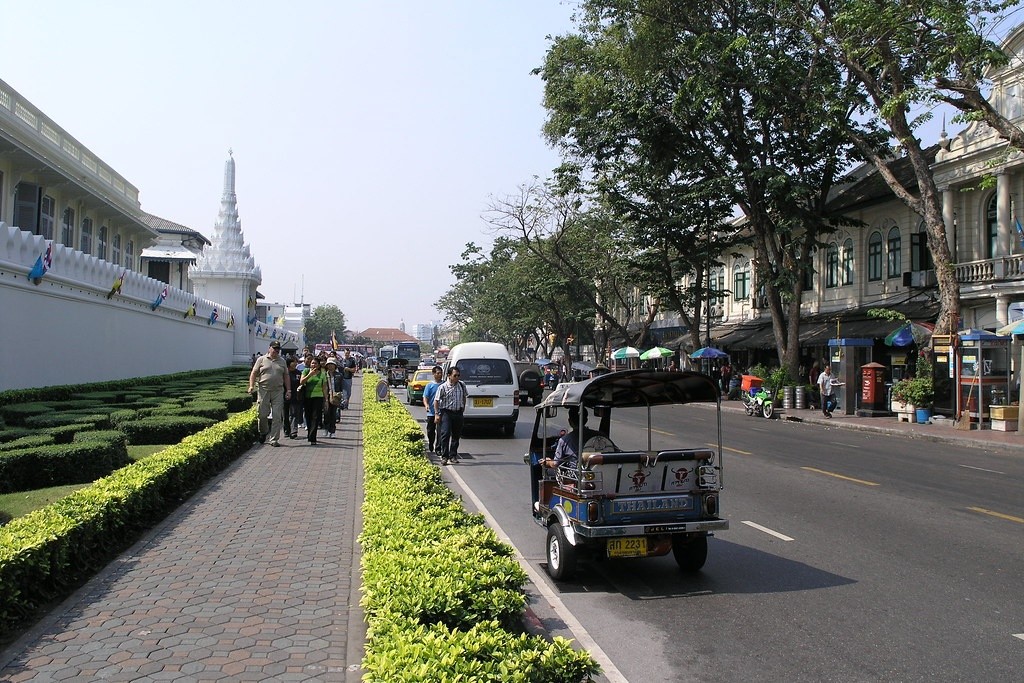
[452,374,460,377]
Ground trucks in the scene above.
[379,346,394,360]
[392,341,421,373]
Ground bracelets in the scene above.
[287,389,291,393]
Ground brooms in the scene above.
[952,367,979,431]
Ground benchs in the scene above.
[553,448,715,502]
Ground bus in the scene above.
[315,344,373,362]
[433,348,451,364]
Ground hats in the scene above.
[270,340,280,348]
[325,358,337,366]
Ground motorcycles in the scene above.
[535,359,560,391]
[741,386,774,419]
[523,368,730,583]
[386,358,409,388]
[375,357,388,373]
[571,361,612,382]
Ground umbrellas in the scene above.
[997,319,1024,335]
[884,319,935,379]
[640,347,675,368]
[612,346,644,370]
[689,346,728,376]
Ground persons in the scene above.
[799,358,829,386]
[248,341,292,446]
[252,352,263,369]
[300,357,328,445]
[423,366,468,464]
[669,360,676,372]
[355,355,362,370]
[534,406,606,511]
[641,361,648,368]
[721,363,733,394]
[283,347,356,440]
[549,363,567,383]
[817,366,837,419]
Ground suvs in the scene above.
[512,361,544,406]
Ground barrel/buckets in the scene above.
[795,386,805,409]
[783,386,794,409]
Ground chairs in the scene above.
[585,436,615,451]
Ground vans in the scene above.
[440,342,520,438]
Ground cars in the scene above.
[404,366,436,406]
[418,352,437,370]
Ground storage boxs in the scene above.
[990,405,1019,431]
[741,375,763,392]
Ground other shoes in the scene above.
[441,456,448,465]
[259,432,267,444]
[284,429,290,437]
[429,444,434,452]
[270,441,279,446]
[290,432,297,439]
[436,450,442,456]
[299,424,302,427]
[323,430,328,436]
[329,433,335,438]
[450,456,459,463]
[534,502,540,510]
[825,412,832,419]
[305,426,307,430]
[311,441,316,445]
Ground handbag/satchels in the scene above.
[330,391,343,406]
[297,385,305,404]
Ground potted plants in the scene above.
[909,379,933,423]
[891,381,916,423]
